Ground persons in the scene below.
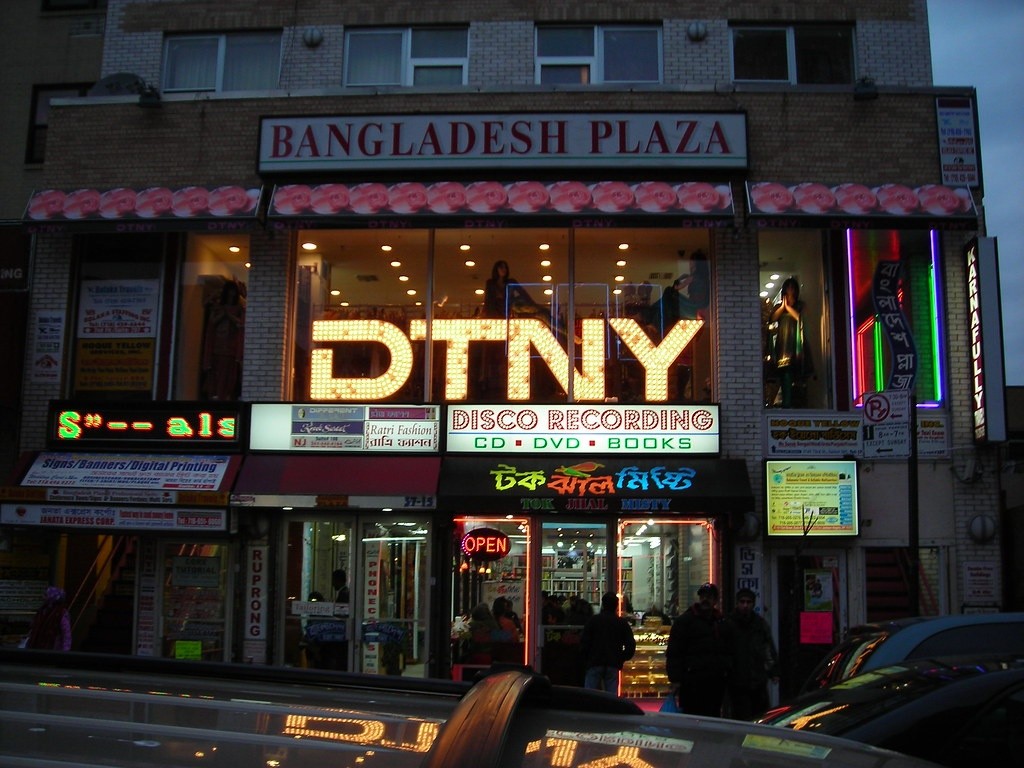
[634,613,640,619]
[25,587,72,652]
[725,588,781,721]
[477,260,583,344]
[624,251,710,334]
[620,595,636,625]
[764,278,815,406]
[558,554,592,570]
[664,581,736,717]
[641,604,671,626]
[807,574,823,598]
[578,591,636,692]
[202,280,246,400]
[459,590,593,686]
[306,569,350,628]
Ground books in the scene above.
[514,555,556,596]
[591,557,632,605]
[552,581,601,604]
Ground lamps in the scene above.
[302,27,324,48]
[968,515,997,546]
[686,21,708,43]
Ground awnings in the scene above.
[435,456,756,515]
[20,450,241,506]
[230,455,440,510]
[18,180,977,222]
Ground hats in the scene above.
[698,583,718,600]
[44,587,66,604]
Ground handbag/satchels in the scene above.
[659,693,678,714]
[766,676,780,708]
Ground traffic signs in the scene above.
[862,389,911,426]
[860,423,912,460]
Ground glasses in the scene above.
[699,591,715,599]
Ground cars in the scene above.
[0,643,945,768]
[748,651,1024,768]
[798,612,1024,699]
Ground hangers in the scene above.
[321,301,628,321]
